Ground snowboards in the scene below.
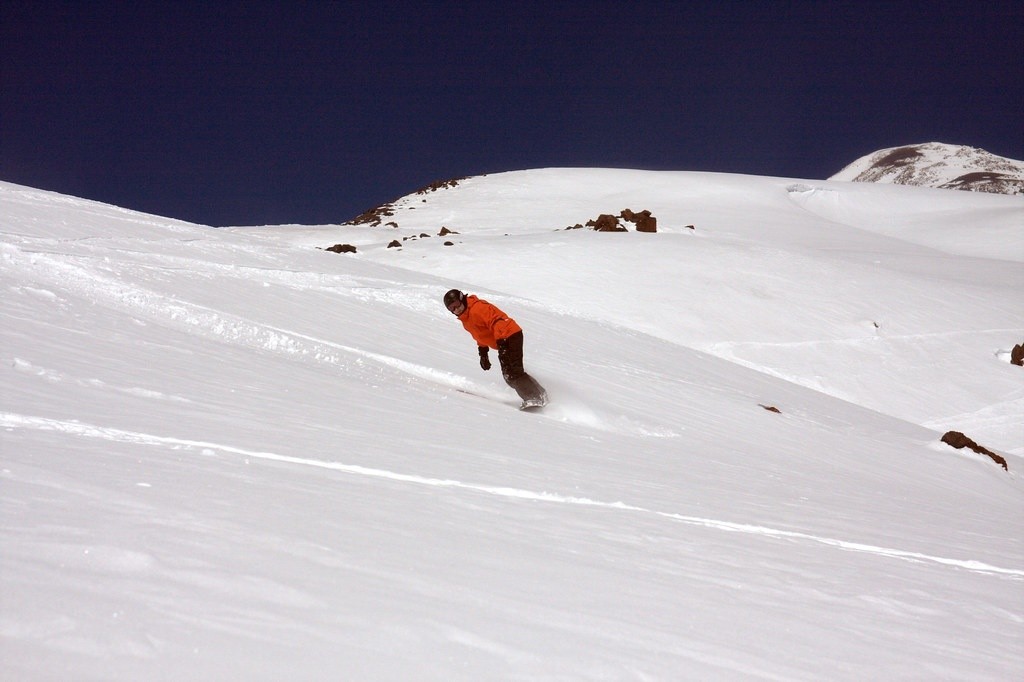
[520,393,549,411]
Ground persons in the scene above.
[444,290,549,410]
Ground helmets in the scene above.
[444,289,466,316]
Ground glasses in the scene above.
[449,301,461,311]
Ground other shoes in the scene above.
[520,392,547,409]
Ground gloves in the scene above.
[497,338,511,364]
[478,347,491,371]
